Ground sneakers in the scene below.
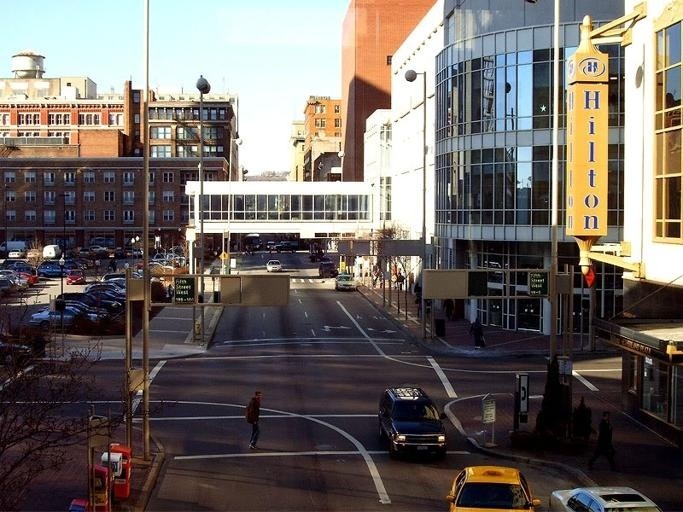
[248,444,258,449]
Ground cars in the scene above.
[550,484,664,511]
[266,259,282,273]
[447,464,541,511]
[244,234,287,252]
[0,236,188,369]
[335,274,357,292]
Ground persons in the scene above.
[442,299,454,321]
[107,258,129,273]
[468,318,482,349]
[244,390,262,449]
[588,410,618,472]
[393,272,407,291]
[378,275,383,289]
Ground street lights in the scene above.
[403,68,429,342]
[196,73,210,342]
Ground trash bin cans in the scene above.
[435,319,446,337]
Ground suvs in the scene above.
[377,383,449,461]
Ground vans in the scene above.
[318,261,337,279]
